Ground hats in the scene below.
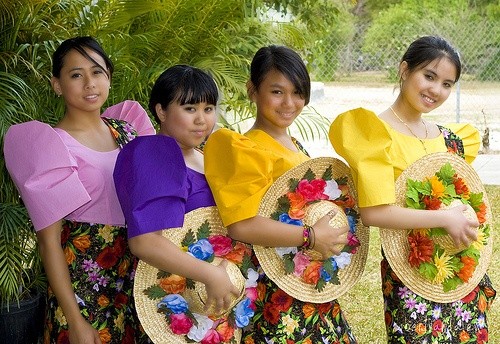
[379,151,493,303]
[252,157,369,303]
[133,205,251,344]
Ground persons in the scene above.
[203,45,357,344]
[113,64,241,344]
[328,36,497,344]
[3,36,156,344]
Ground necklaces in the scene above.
[390,106,428,156]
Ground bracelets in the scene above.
[299,224,315,250]
